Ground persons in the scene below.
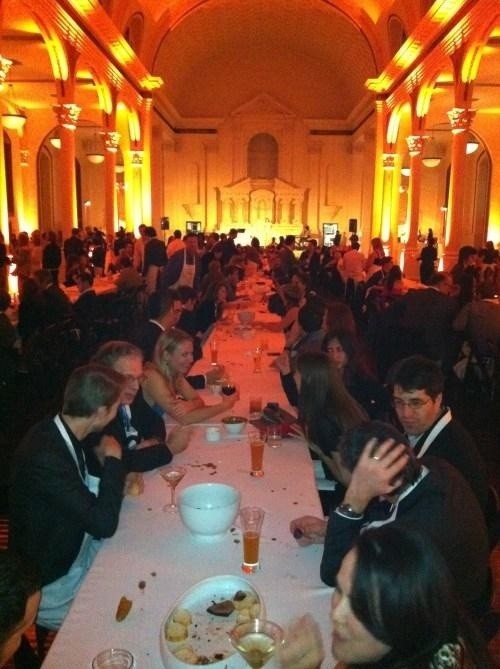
[1,341,192,637]
[1,224,238,449]
[269,235,499,669]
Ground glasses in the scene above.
[392,397,431,409]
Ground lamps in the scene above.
[466,134,479,155]
[422,123,443,169]
[86,133,105,165]
[48,137,60,150]
[0,68,26,128]
[401,158,410,177]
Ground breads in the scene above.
[165,591,260,666]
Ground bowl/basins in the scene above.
[175,483,241,540]
[237,311,255,324]
[208,378,227,395]
[220,416,247,435]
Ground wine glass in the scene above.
[156,465,185,514]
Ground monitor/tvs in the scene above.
[186,221,201,235]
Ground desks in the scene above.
[40,244,350,669]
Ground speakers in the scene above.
[160,217,169,230]
[349,218,357,232]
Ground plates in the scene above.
[157,572,267,668]
[232,325,255,336]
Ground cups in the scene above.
[228,618,283,669]
[205,426,220,442]
[249,344,265,374]
[239,506,265,576]
[248,430,268,479]
[249,394,261,415]
[264,420,283,449]
[208,340,220,365]
[221,380,235,395]
[90,647,133,669]
